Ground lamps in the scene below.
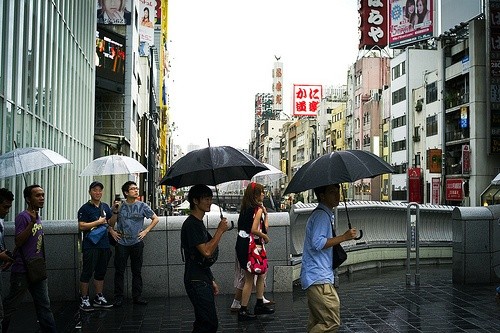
[433,21,467,41]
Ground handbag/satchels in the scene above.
[246,241,267,274]
[192,232,219,269]
[86,226,107,244]
[332,230,347,270]
[26,257,48,291]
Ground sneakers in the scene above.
[80,296,94,311]
[94,294,113,308]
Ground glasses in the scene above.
[128,187,139,190]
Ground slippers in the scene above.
[264,301,275,305]
[231,308,248,311]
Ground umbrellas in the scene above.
[157,138,270,232]
[0,141,73,222]
[79,154,149,208]
[281,141,396,239]
[215,161,285,192]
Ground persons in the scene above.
[403,0,418,33]
[300,183,357,333]
[140,7,153,28]
[230,182,276,319]
[78,181,121,313]
[108,182,159,306]
[0,185,57,333]
[180,185,228,333]
[97,0,131,25]
[160,199,180,216]
[416,0,430,29]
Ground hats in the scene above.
[90,182,103,190]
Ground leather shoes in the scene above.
[238,308,256,320]
[113,300,123,307]
[132,300,147,306]
[255,304,275,314]
